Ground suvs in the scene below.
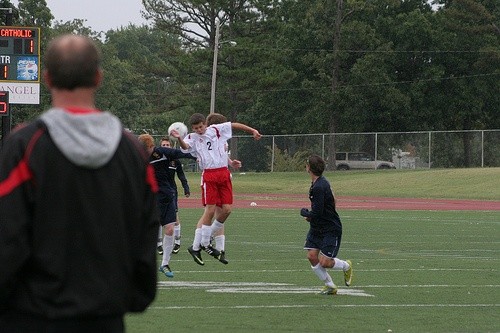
[325,152,397,170]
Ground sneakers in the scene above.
[343,260,352,285]
[213,251,228,265]
[319,286,338,295]
[159,265,173,278]
[172,242,180,253]
[188,246,204,265]
[199,243,220,256]
[158,245,164,254]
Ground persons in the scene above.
[131,112,263,281]
[301,155,353,296]
[0,35,163,333]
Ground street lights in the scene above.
[210,40,236,114]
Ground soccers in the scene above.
[167,122,188,140]
